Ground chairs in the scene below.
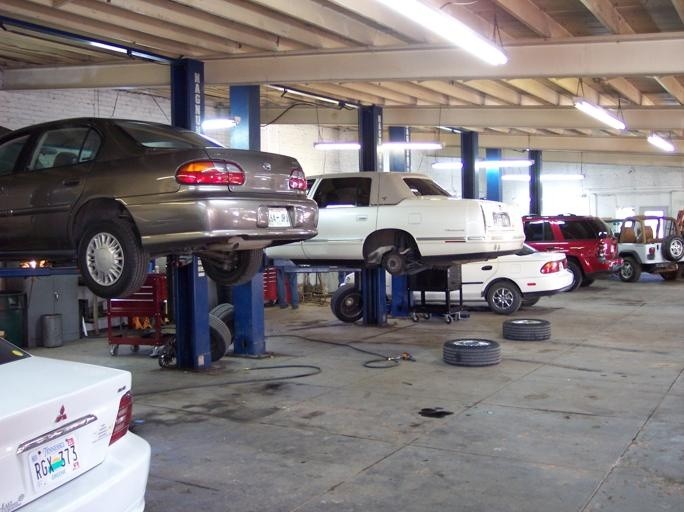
[619,227,652,243]
[34,152,78,169]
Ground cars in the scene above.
[260,171,526,275]
[0,117,320,299]
[338,240,573,314]
[0,336,151,512]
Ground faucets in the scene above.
[52,291,62,303]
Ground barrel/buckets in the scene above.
[41,312,64,349]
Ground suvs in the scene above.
[521,214,624,292]
[602,216,684,282]
